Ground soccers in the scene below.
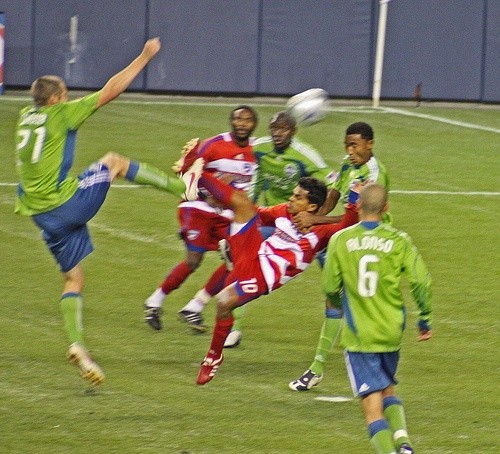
[288,86,330,125]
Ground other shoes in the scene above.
[143,299,162,331]
[175,307,208,333]
[171,137,201,173]
[66,341,106,387]
[179,156,205,201]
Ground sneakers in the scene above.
[223,330,243,348]
[288,369,324,391]
[196,352,224,385]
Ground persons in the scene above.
[144,106,259,333]
[320,182,433,454]
[289,121,392,392]
[14,38,205,385]
[171,136,374,384]
[222,110,335,348]
